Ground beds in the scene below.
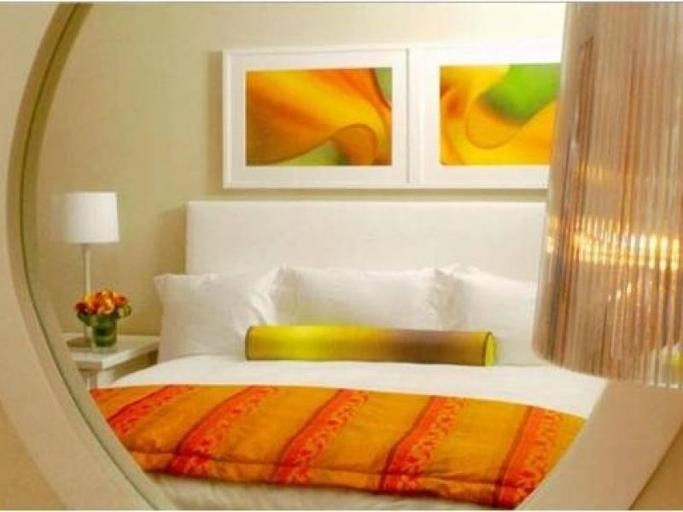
[86,202,610,508]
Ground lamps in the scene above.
[62,193,119,292]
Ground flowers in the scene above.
[76,288,132,318]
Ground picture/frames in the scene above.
[220,46,562,189]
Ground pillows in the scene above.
[245,325,496,366]
[152,272,275,366]
[429,266,540,365]
[276,263,435,331]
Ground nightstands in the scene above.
[62,332,160,389]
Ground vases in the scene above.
[90,320,117,347]
